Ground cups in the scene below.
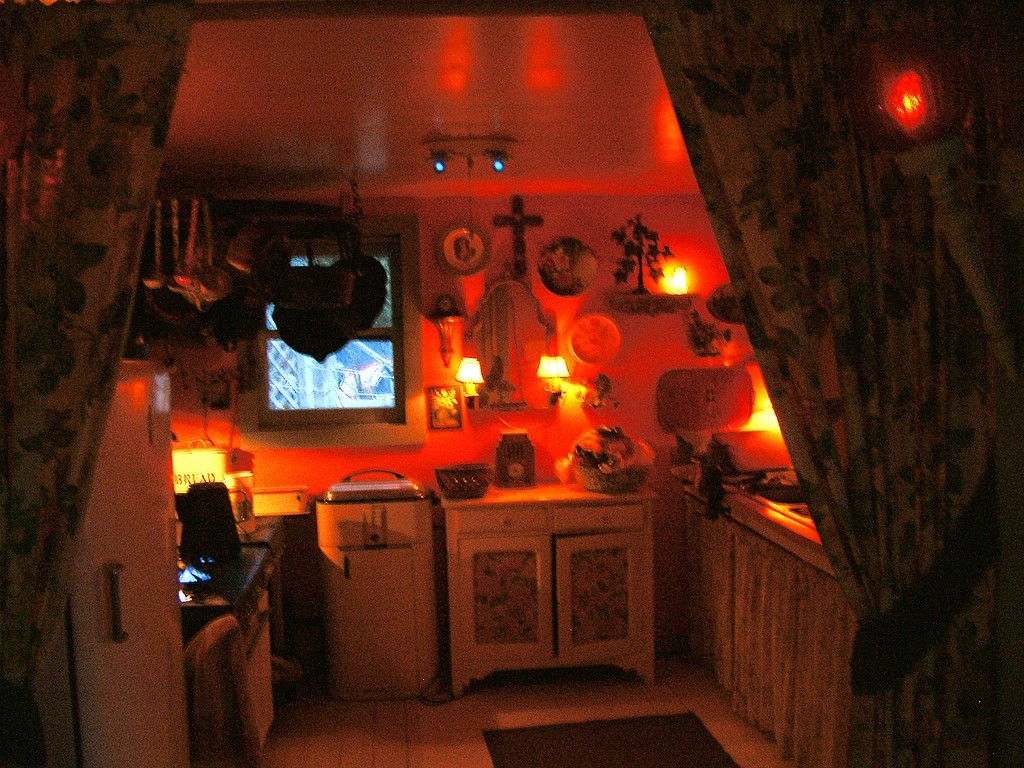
[226,471,256,535]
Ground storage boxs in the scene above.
[251,484,309,515]
[169,438,240,494]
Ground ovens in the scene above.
[234,590,276,751]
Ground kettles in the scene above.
[174,482,250,557]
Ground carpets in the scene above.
[481,711,740,768]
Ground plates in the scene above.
[538,238,597,296]
[568,313,621,366]
[435,224,489,274]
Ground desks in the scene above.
[174,512,289,751]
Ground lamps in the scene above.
[122,332,153,368]
[454,357,485,411]
[673,266,691,312]
[535,354,571,408]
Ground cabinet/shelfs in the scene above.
[440,481,664,698]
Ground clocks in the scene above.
[566,312,624,368]
[437,295,458,316]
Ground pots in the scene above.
[335,222,387,328]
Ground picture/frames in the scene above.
[427,381,465,431]
[434,222,491,277]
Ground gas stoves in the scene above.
[176,545,271,609]
